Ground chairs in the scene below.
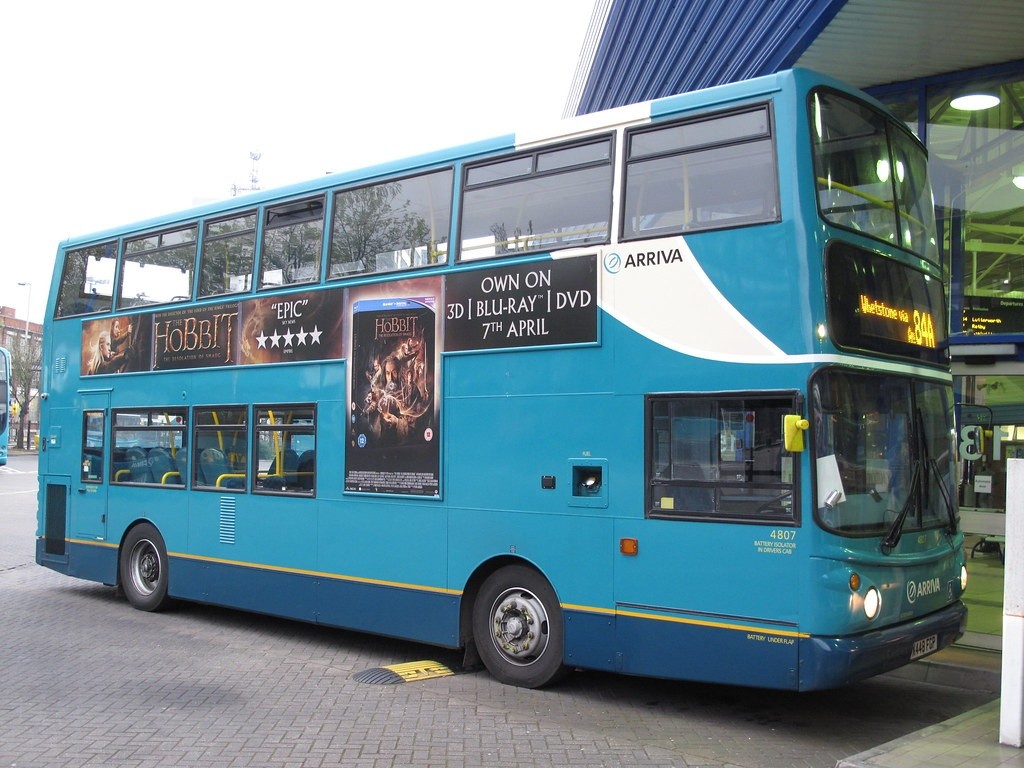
[174,446,206,485]
[126,447,154,483]
[147,448,181,484]
[200,448,243,488]
[267,449,299,486]
[295,449,314,490]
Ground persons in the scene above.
[361,336,429,441]
[87,319,134,374]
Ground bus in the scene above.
[36,68,972,691]
[0,346,13,467]
